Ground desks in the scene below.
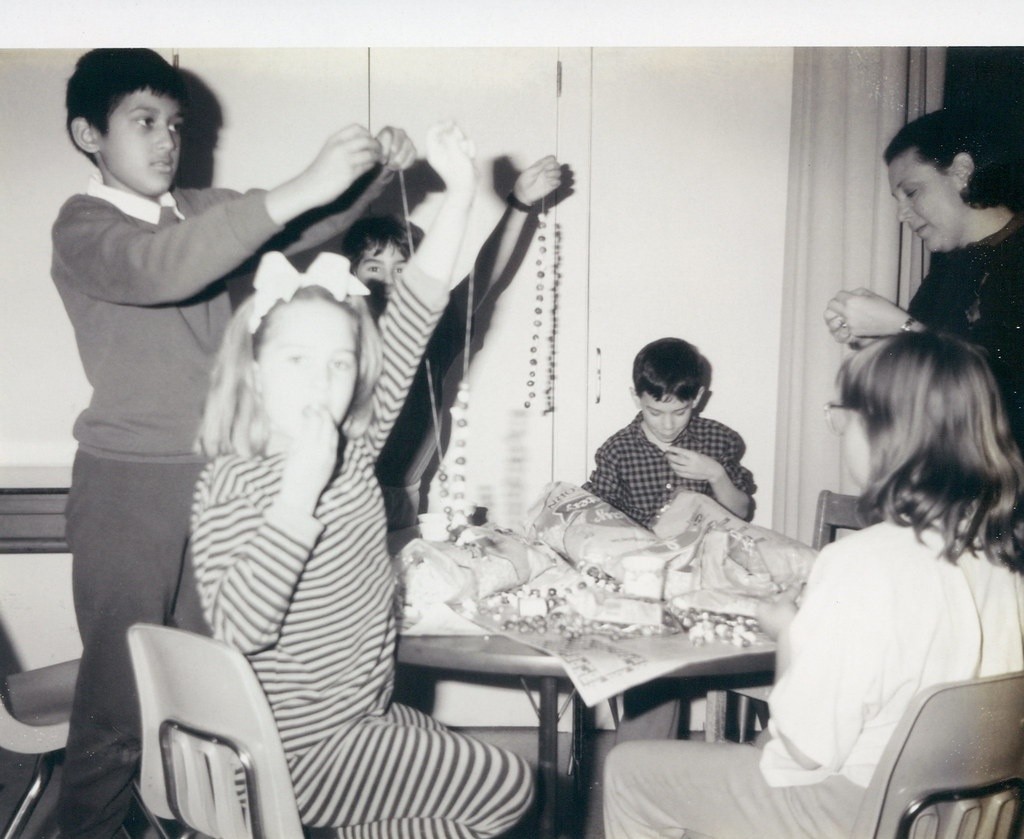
[398,636,778,839]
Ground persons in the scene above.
[342,154,562,533]
[823,111,1024,543]
[582,338,755,746]
[50,48,418,839]
[603,333,1024,839]
[190,119,534,839]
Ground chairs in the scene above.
[705,489,865,741]
[848,671,1024,839]
[0,657,174,839]
[127,619,309,839]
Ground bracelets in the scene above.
[900,317,916,333]
[506,192,534,213]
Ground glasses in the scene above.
[823,402,870,436]
